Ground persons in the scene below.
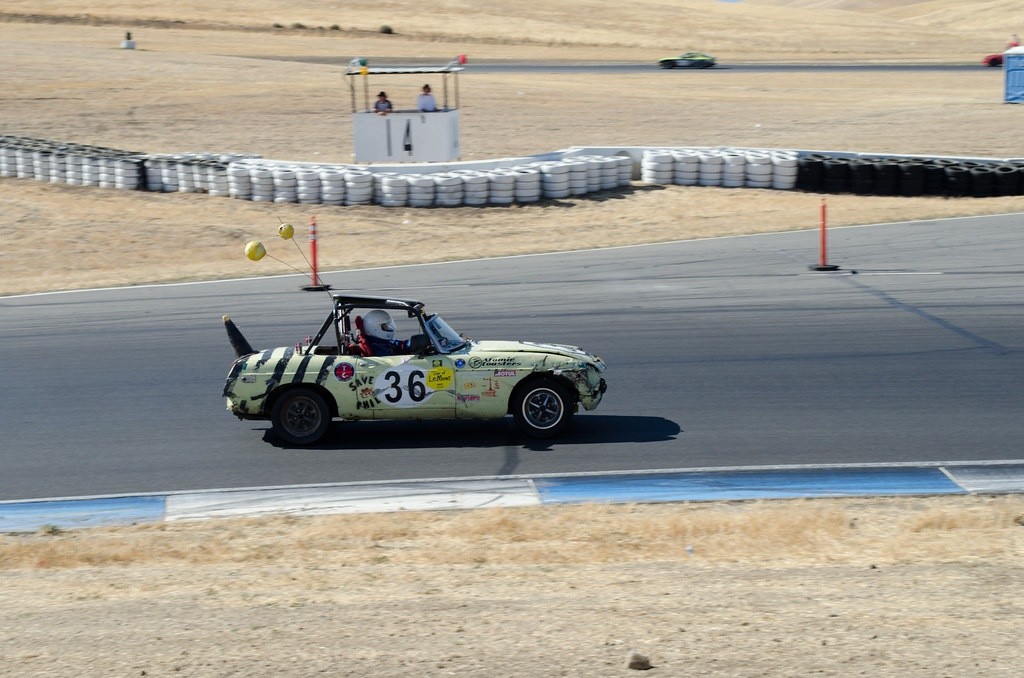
[371,92,393,116]
[361,310,429,356]
[416,83,438,112]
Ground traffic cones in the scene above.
[806,198,843,273]
[301,214,331,293]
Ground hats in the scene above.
[378,92,387,97]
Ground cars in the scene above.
[220,295,608,439]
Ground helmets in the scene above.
[363,310,396,340]
